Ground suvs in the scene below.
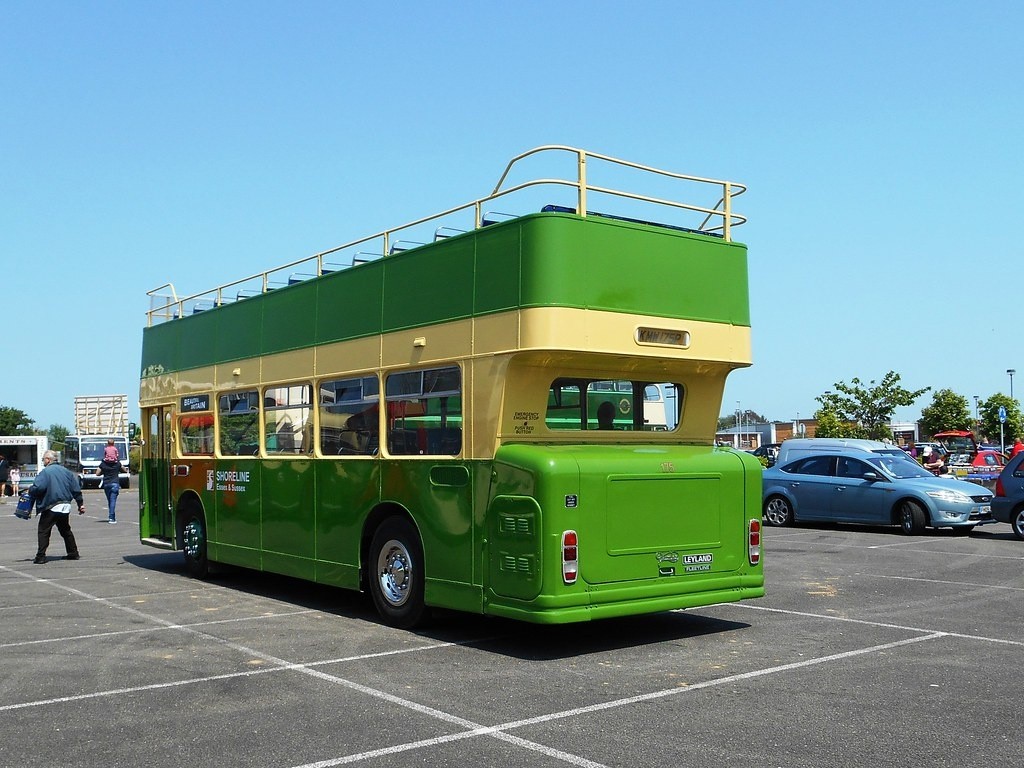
[989,450,1024,539]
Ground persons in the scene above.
[882,437,1024,475]
[95,439,127,524]
[717,438,724,447]
[27,449,85,564]
[0,456,20,497]
[597,401,616,430]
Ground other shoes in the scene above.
[34,555,49,564]
[12,494,15,497]
[62,555,79,560]
[109,519,118,524]
[16,494,18,496]
[1,495,8,498]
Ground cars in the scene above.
[762,451,999,535]
[934,431,1010,495]
[746,443,780,467]
[899,442,1014,463]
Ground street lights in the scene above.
[736,400,742,447]
[745,410,750,448]
[1007,369,1016,397]
[972,395,979,432]
[797,411,800,437]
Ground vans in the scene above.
[777,439,925,469]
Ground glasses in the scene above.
[41,458,48,461]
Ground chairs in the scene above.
[861,464,875,473]
[235,432,461,455]
[173,211,519,319]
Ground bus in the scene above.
[129,145,765,625]
[396,382,669,433]
[63,434,129,489]
[168,384,424,520]
